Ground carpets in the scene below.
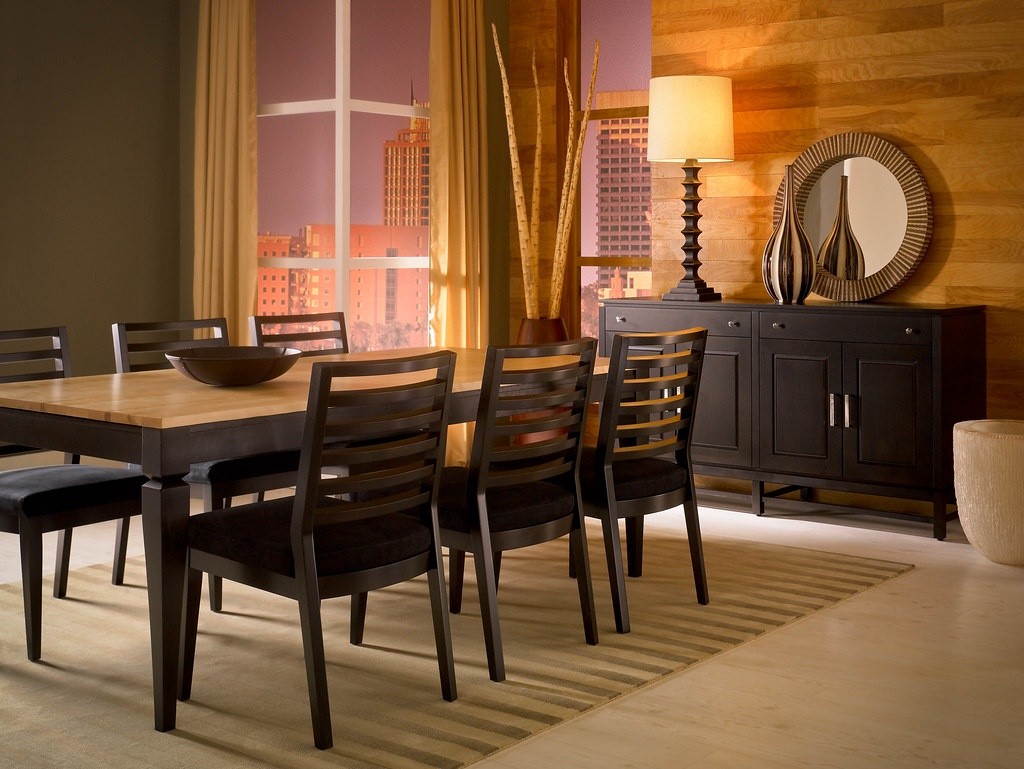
[0,522,910,769]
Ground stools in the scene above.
[951,420,1024,568]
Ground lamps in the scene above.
[648,76,736,301]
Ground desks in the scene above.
[0,347,652,730]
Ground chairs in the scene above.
[0,314,709,665]
[178,345,458,749]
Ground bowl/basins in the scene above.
[164,346,303,386]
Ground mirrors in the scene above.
[770,133,932,301]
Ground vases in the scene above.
[761,165,815,303]
[813,174,865,280]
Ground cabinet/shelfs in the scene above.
[598,295,986,541]
